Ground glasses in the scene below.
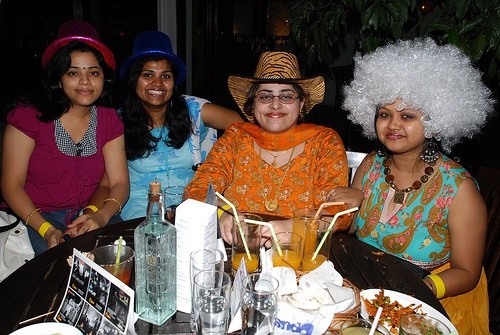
[273,34,288,40]
[252,92,302,104]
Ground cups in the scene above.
[338,318,391,335]
[290,207,321,258]
[302,219,334,274]
[231,212,263,277]
[240,273,279,335]
[270,231,302,284]
[398,314,451,335]
[164,186,190,224]
[87,245,136,285]
[190,248,231,335]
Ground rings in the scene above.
[330,195,333,198]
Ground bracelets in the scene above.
[426,274,445,299]
[102,198,122,216]
[38,222,53,238]
[424,276,438,297]
[26,208,41,225]
[79,204,98,217]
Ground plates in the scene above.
[9,322,83,335]
[359,289,459,335]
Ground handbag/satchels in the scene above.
[0,208,36,282]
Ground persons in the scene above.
[0,20,130,258]
[326,38,497,335]
[183,52,354,245]
[60,31,245,243]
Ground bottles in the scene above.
[134,182,178,327]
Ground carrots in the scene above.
[361,288,421,327]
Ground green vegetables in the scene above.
[374,297,444,335]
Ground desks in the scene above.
[0,213,451,335]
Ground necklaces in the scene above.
[259,147,296,211]
[383,150,436,204]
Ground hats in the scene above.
[227,52,324,123]
[120,31,186,87]
[41,20,116,71]
